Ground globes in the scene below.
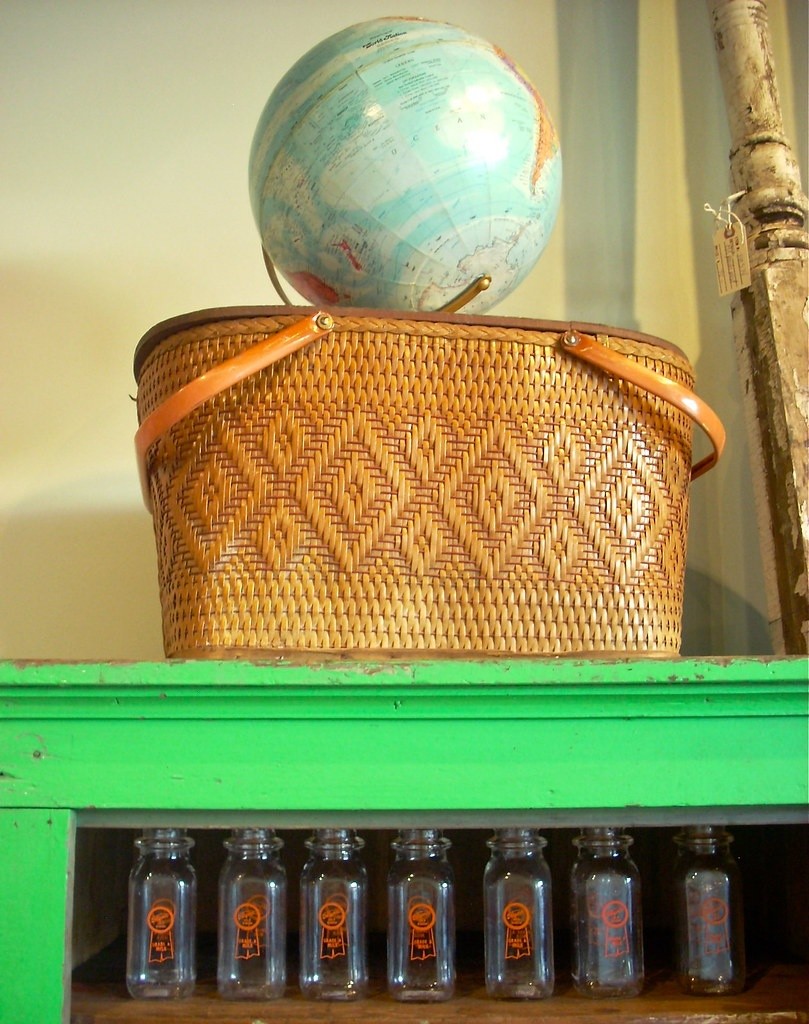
[244,12,564,314]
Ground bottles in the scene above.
[216,829,288,999]
[386,826,457,1002]
[298,827,372,1001]
[670,825,748,995]
[567,826,646,995]
[125,830,199,999]
[483,827,557,999]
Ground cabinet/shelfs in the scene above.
[0,652,809,1024]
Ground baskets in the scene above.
[134,305,727,659]
